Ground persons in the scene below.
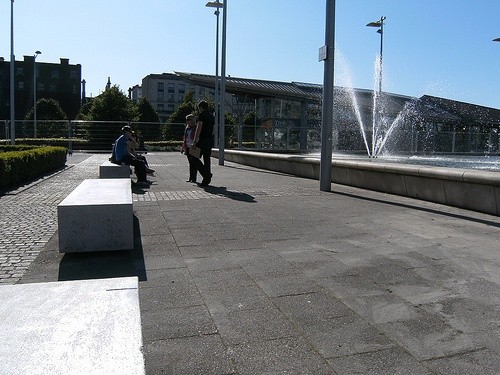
[180,113,213,183]
[112,130,155,184]
[116,126,154,185]
[189,101,213,188]
[129,132,142,157]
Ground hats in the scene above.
[121,126,134,133]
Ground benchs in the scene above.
[57,178,134,253]
[99,161,130,178]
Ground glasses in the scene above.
[186,119,191,121]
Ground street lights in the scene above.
[366,16,386,158]
[205,0,224,148]
[32,51,44,141]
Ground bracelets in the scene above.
[192,140,198,144]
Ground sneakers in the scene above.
[138,181,153,187]
[199,173,212,187]
[186,179,196,183]
[146,169,154,175]
